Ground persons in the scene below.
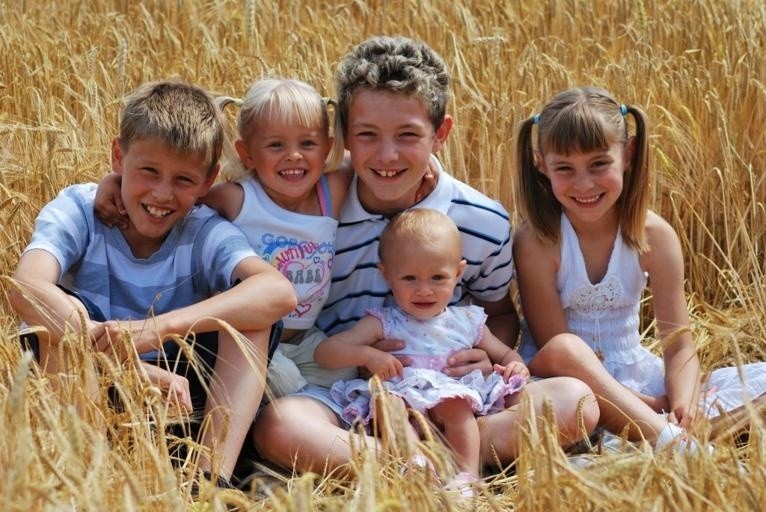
[514,86,766,474]
[253,35,600,482]
[93,77,439,399]
[314,209,531,497]
[8,82,298,510]
